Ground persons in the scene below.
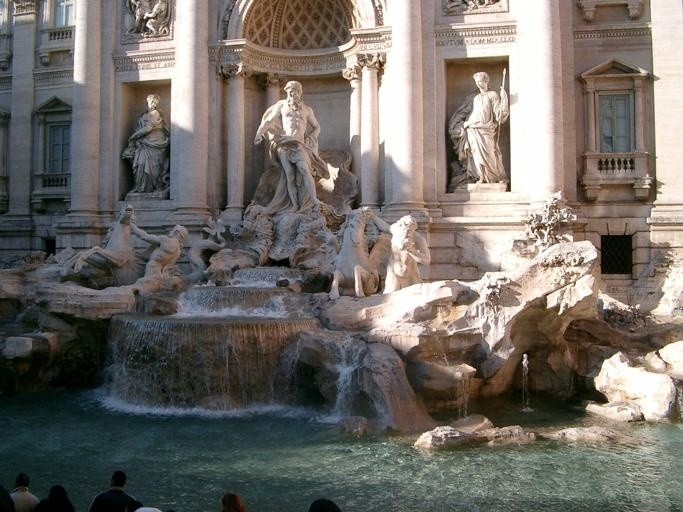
[448,71,509,183]
[129,0,169,37]
[0,473,75,512]
[129,93,168,192]
[375,215,431,293]
[253,81,321,212]
[131,224,190,284]
[222,493,246,512]
[309,498,340,512]
[87,470,136,512]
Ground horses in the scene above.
[57,202,146,286]
[322,204,390,304]
